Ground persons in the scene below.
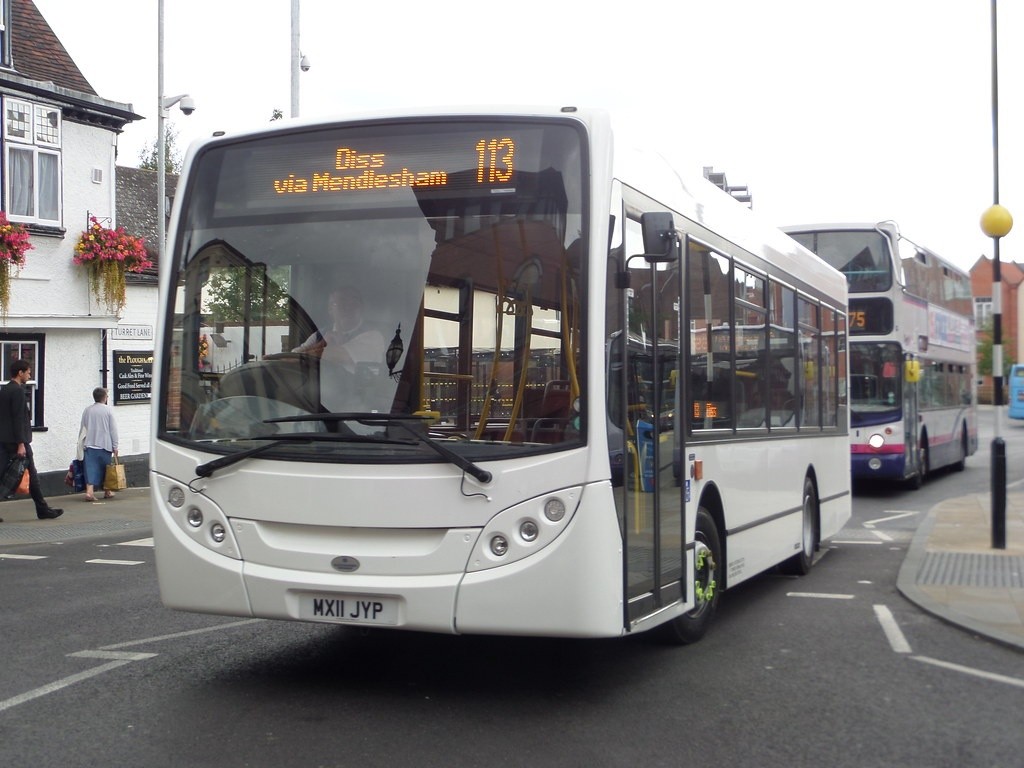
[288,289,384,374]
[0,359,64,520]
[79,388,118,501]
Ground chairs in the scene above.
[530,379,573,445]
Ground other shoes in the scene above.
[104,492,115,498]
[0,518,3,522]
[37,508,63,519]
[85,494,98,501]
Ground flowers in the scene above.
[198,334,209,368]
[0,210,35,333]
[72,215,152,319]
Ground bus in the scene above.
[1007,364,1024,420]
[849,374,878,404]
[150,107,852,646]
[776,220,979,491]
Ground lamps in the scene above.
[386,322,411,383]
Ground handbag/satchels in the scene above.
[0,455,29,500]
[64,459,87,492]
[103,455,126,489]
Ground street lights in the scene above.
[291,52,312,119]
[981,205,1013,406]
[156,93,196,295]
[689,241,713,431]
